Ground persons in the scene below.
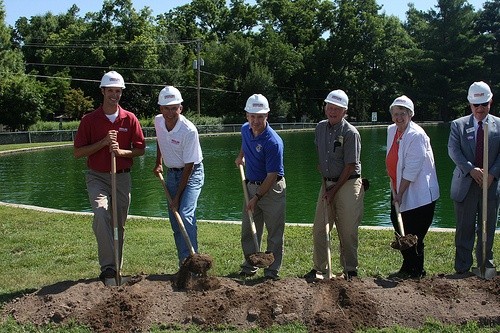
[447,81,500,275]
[385,94,441,280]
[72,69,147,290]
[235,94,287,281]
[151,84,206,272]
[303,89,366,281]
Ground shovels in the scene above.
[390,181,418,250]
[471,122,496,281]
[238,157,275,269]
[158,171,212,274]
[105,150,132,286]
[315,176,337,281]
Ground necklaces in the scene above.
[396,127,408,144]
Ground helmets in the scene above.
[157,86,183,105]
[324,89,348,110]
[99,71,126,89]
[244,94,270,114]
[390,94,414,117]
[467,81,493,104]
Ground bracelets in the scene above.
[103,137,108,147]
[255,194,262,201]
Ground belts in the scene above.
[250,176,282,185]
[109,168,131,174]
[325,174,360,181]
[168,168,183,171]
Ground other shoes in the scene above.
[99,268,116,279]
[390,270,426,279]
[239,271,256,277]
[447,271,470,279]
[265,274,280,280]
[344,271,357,280]
[304,269,326,278]
